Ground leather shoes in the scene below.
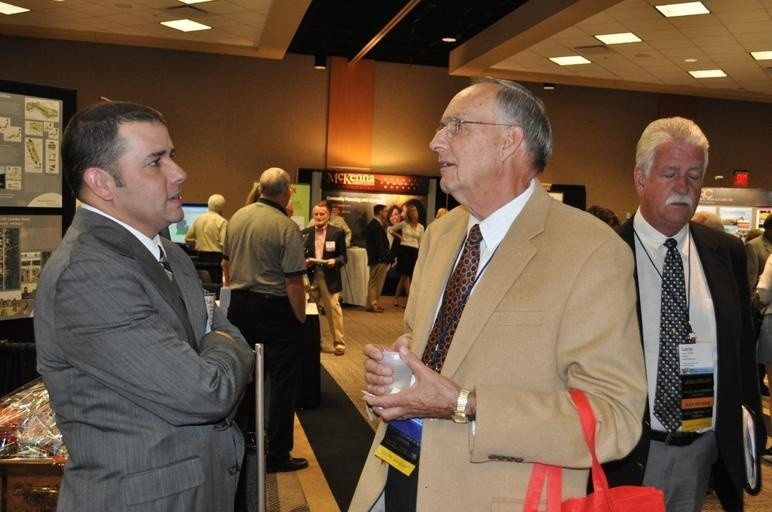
[266,456,308,473]
[334,343,346,355]
[366,304,384,312]
[761,385,769,396]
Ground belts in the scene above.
[231,289,288,300]
[199,251,222,256]
[315,264,324,267]
[649,430,706,447]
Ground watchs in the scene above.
[454,387,469,424]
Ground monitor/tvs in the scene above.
[167,203,221,244]
[0,80,78,215]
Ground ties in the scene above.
[421,224,483,374]
[157,245,179,291]
[653,238,695,433]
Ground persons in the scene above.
[693,210,725,234]
[245,182,294,218]
[746,228,763,242]
[222,167,308,471]
[390,206,424,308]
[436,207,449,220]
[366,205,391,312]
[32,101,255,511]
[745,214,771,324]
[186,193,229,265]
[600,118,765,512]
[300,202,349,356]
[756,253,771,460]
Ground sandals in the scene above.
[761,446,772,466]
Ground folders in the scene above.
[736,400,762,495]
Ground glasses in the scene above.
[382,210,387,213]
[437,118,513,139]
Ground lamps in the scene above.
[313,46,327,69]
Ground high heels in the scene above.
[403,304,406,308]
[393,296,399,306]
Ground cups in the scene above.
[379,352,412,395]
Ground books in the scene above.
[739,403,764,494]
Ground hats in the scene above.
[758,212,772,229]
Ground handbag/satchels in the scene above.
[524,392,665,512]
